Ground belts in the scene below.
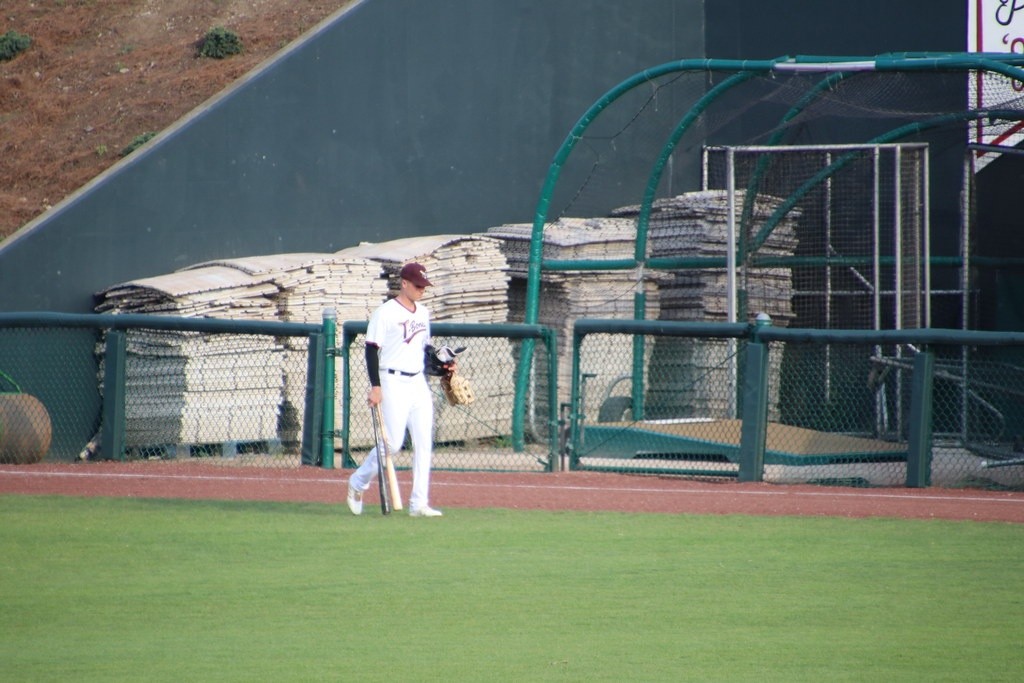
[389,369,420,376]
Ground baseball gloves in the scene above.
[440,370,479,407]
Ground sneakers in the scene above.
[409,500,442,516]
[347,484,362,515]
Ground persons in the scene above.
[347,263,455,517]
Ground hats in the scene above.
[400,262,434,286]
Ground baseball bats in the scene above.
[376,403,404,512]
[371,406,391,516]
